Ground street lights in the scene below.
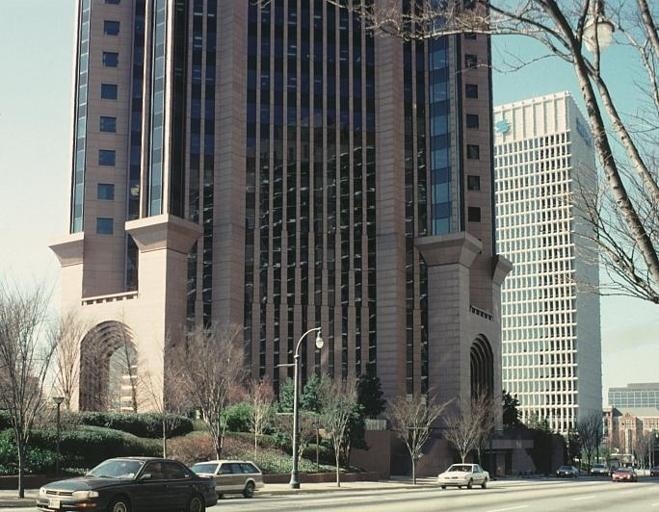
[579,1,659,59]
[53,396,64,481]
[596,421,608,463]
[648,430,659,469]
[289,326,324,489]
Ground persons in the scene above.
[650,466,653,473]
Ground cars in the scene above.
[190,459,264,499]
[36,456,219,512]
[437,463,490,489]
[556,456,659,482]
